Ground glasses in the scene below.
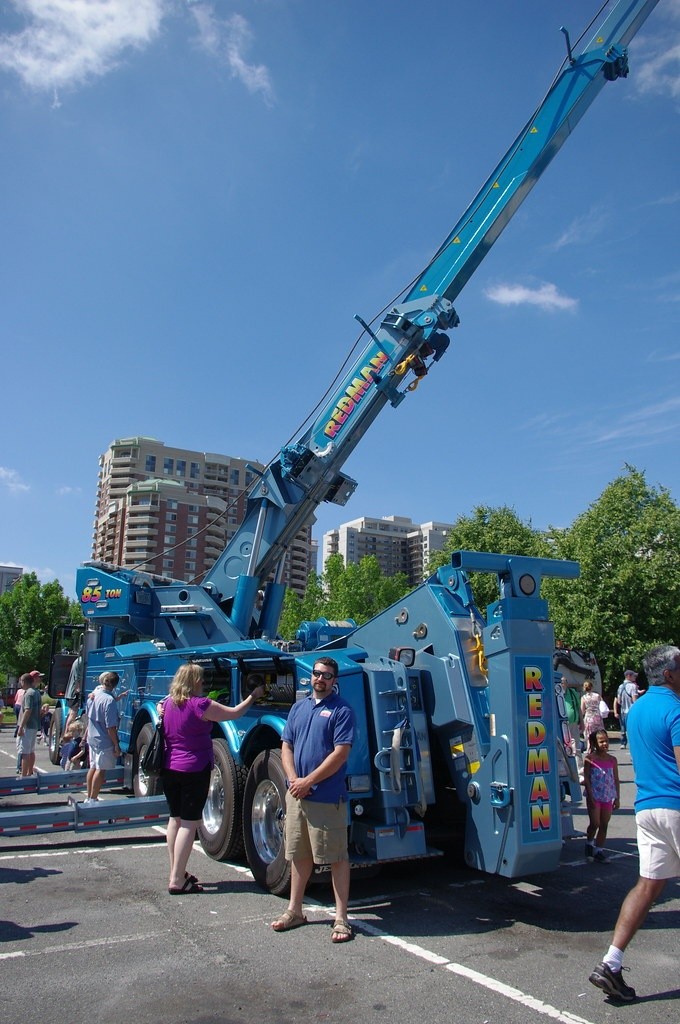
[313,670,335,680]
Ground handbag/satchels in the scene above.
[141,699,169,775]
[598,696,610,718]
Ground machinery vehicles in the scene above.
[47,0,657,899]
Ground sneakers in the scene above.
[589,960,636,1000]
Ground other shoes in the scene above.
[593,850,611,863]
[84,798,106,803]
[15,775,37,780]
[620,745,626,749]
[584,842,595,858]
[15,768,35,774]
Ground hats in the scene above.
[30,671,46,677]
[625,670,639,677]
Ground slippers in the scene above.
[272,909,309,931]
[331,920,352,942]
[168,879,204,895]
[184,871,200,883]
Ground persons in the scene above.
[582,728,621,862]
[59,672,130,802]
[272,656,354,941]
[156,664,264,894]
[562,678,584,753]
[580,681,604,748]
[13,670,53,775]
[589,645,680,1000]
[0,695,4,721]
[613,669,645,749]
